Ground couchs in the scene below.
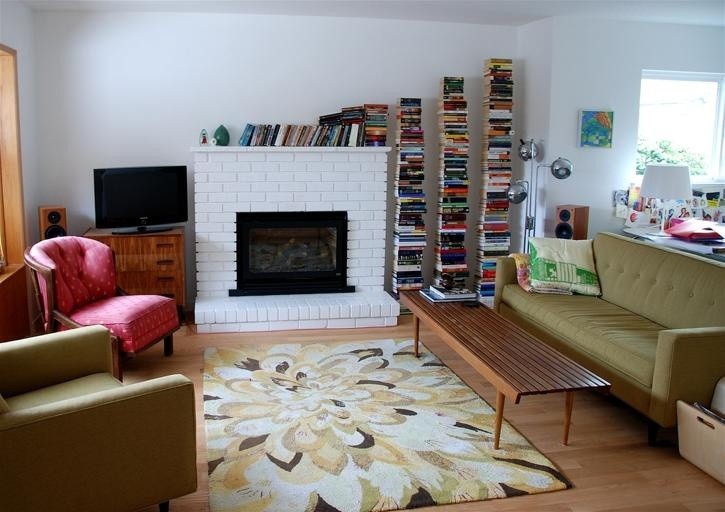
[493,232,724,447]
[0,325,198,512]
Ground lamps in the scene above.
[507,138,574,253]
[640,164,692,239]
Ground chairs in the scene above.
[25,236,180,375]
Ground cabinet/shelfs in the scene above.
[82,227,185,321]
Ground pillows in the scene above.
[528,238,601,296]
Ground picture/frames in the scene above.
[577,108,616,150]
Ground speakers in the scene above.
[555,204,590,240]
[37,206,67,241]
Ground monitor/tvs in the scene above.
[93,165,188,236]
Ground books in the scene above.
[239,105,364,146]
[473,58,514,296]
[433,76,471,288]
[420,288,477,303]
[430,285,476,298]
[392,98,428,293]
[363,104,388,146]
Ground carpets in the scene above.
[204,338,571,512]
